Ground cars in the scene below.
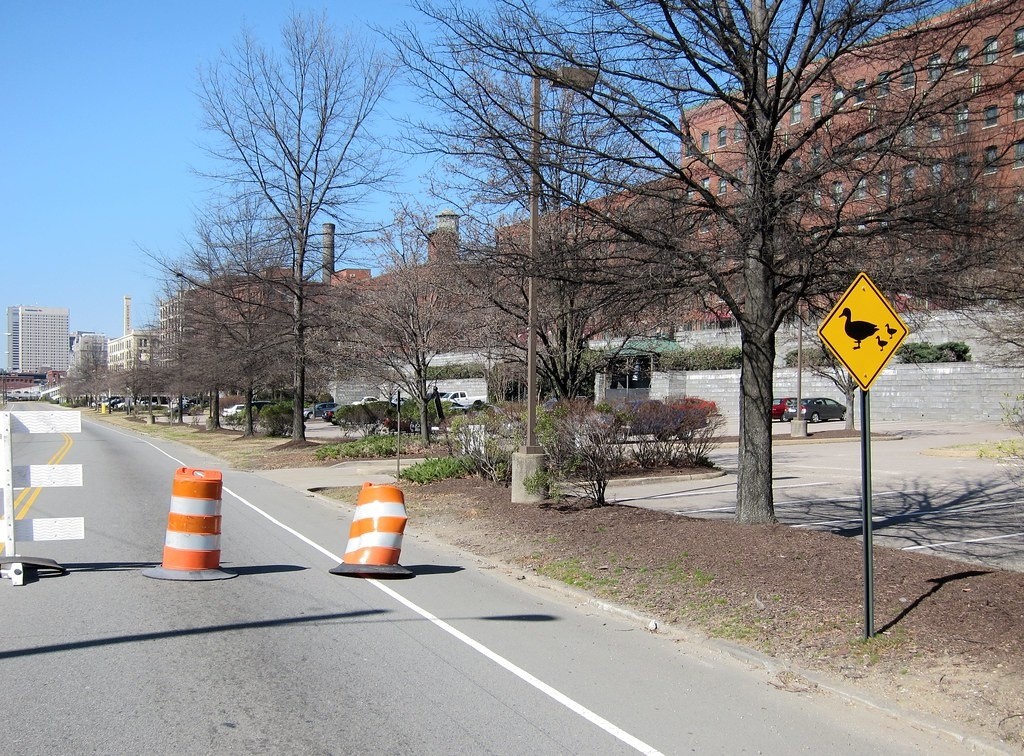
[427,392,453,405]
[248,401,294,427]
[304,403,340,419]
[369,401,397,411]
[222,404,259,422]
[351,396,380,406]
[391,397,409,405]
[772,398,800,422]
[513,396,602,435]
[97,395,219,417]
[585,400,708,445]
[303,408,315,420]
[785,397,847,423]
[322,404,386,426]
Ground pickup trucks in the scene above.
[440,390,488,414]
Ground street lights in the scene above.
[3,351,11,405]
[517,63,601,453]
